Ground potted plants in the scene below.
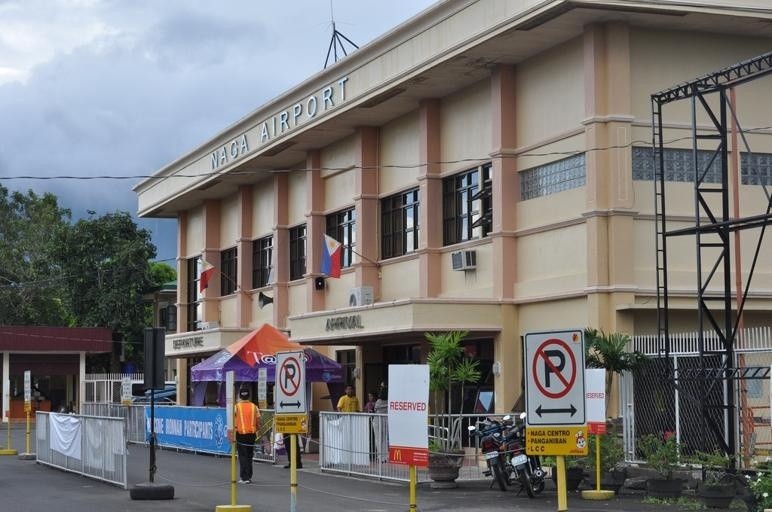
[638,429,736,509]
[549,327,651,494]
[423,329,481,488]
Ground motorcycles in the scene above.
[465,414,511,489]
[499,413,547,498]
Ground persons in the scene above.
[281,432,303,470]
[336,383,361,413]
[363,388,378,458]
[371,386,390,465]
[233,387,262,485]
[379,379,389,390]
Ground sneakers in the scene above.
[284,462,302,468]
[238,477,250,485]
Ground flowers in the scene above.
[745,459,771,511]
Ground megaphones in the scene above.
[314,277,325,289]
[257,291,273,309]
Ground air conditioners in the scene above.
[345,287,374,307]
[196,322,218,330]
[451,249,476,271]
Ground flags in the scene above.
[198,257,217,295]
[319,232,344,279]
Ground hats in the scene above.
[240,388,249,397]
